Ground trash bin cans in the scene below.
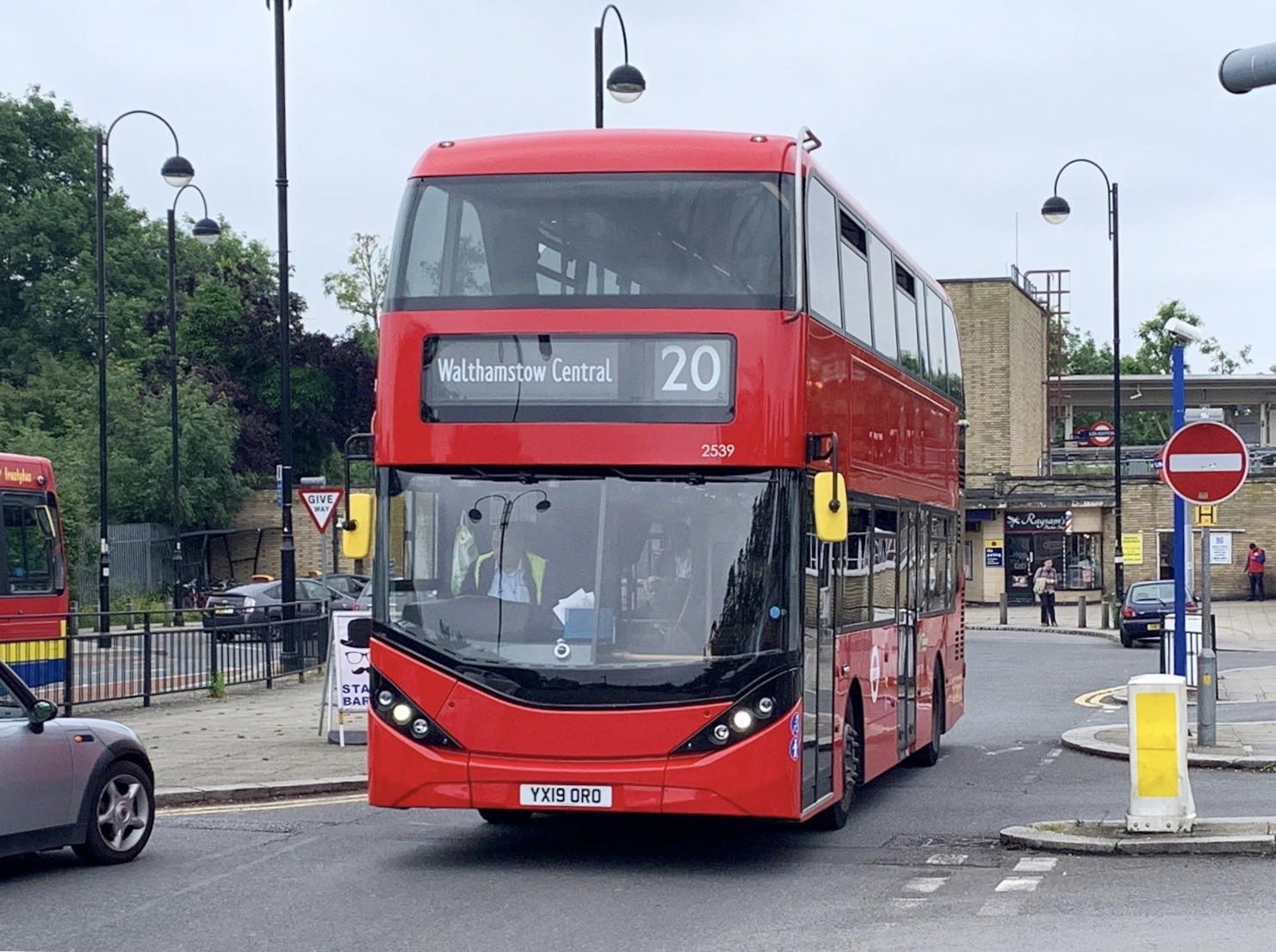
[1125,674,1197,833]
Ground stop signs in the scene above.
[1161,419,1248,506]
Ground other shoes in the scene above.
[1041,622,1047,626]
[1259,596,1264,601]
[1246,597,1255,601]
[1052,621,1058,627]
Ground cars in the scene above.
[354,576,404,610]
[202,582,356,640]
[1119,580,1202,648]
[312,573,373,597]
[1,662,157,871]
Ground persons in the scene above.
[1033,558,1059,627]
[461,528,551,607]
[1242,543,1265,602]
[633,522,694,639]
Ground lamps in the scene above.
[1129,387,1142,401]
[1201,389,1207,399]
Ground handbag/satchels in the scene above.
[449,510,479,595]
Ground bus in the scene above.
[340,125,966,827]
[1,453,70,698]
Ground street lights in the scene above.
[95,110,193,640]
[1040,157,1124,629]
[593,3,645,126]
[165,185,222,621]
